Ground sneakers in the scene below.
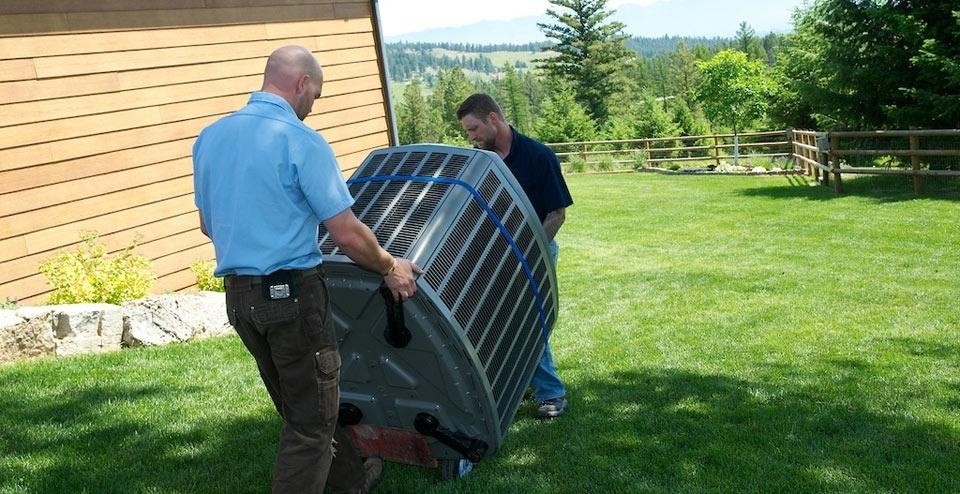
[357,458,382,493]
[538,397,567,418]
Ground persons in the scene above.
[193,42,425,494]
[455,91,571,418]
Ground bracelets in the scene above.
[383,257,397,277]
